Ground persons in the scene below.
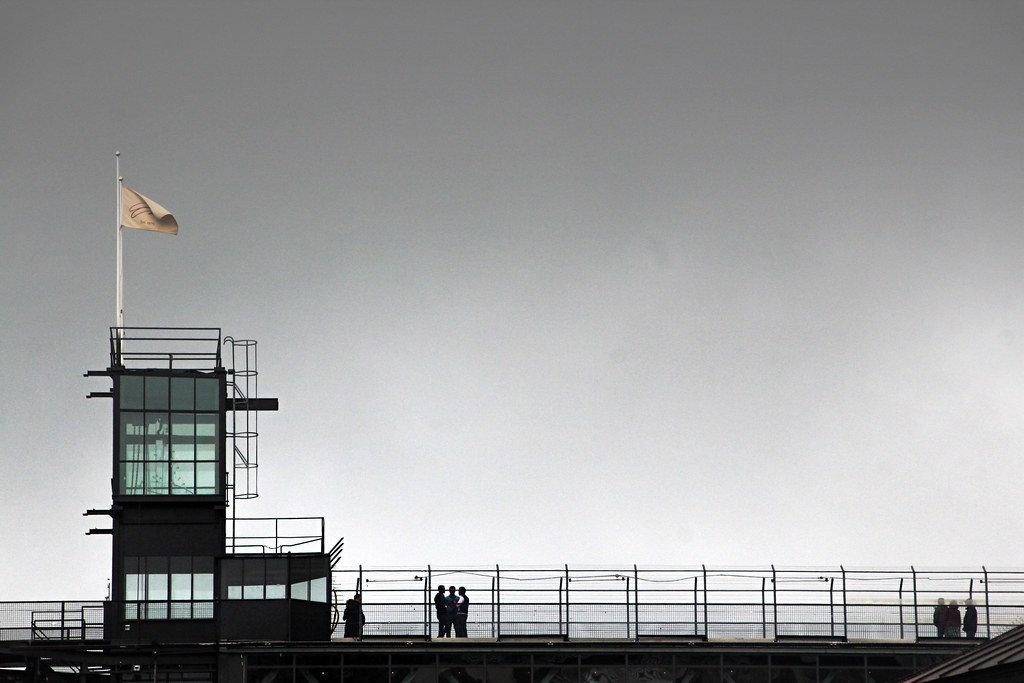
[945,599,961,637]
[444,586,460,638]
[434,585,452,638]
[343,594,365,638]
[934,598,948,638]
[454,587,469,637]
[962,598,977,638]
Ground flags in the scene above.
[121,186,178,236]
